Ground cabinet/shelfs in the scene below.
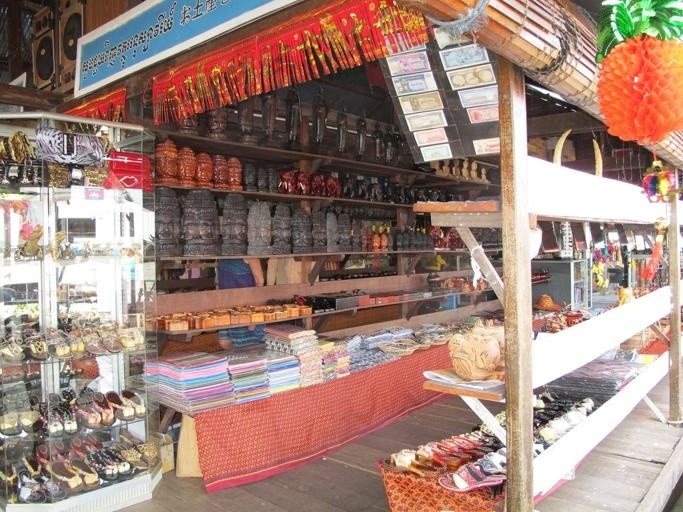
[0,112,682,511]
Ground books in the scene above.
[135,324,350,413]
[423,365,507,392]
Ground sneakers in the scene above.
[0,323,146,362]
[1,389,146,439]
[0,429,160,502]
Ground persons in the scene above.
[177,256,320,290]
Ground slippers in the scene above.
[391,388,602,492]
